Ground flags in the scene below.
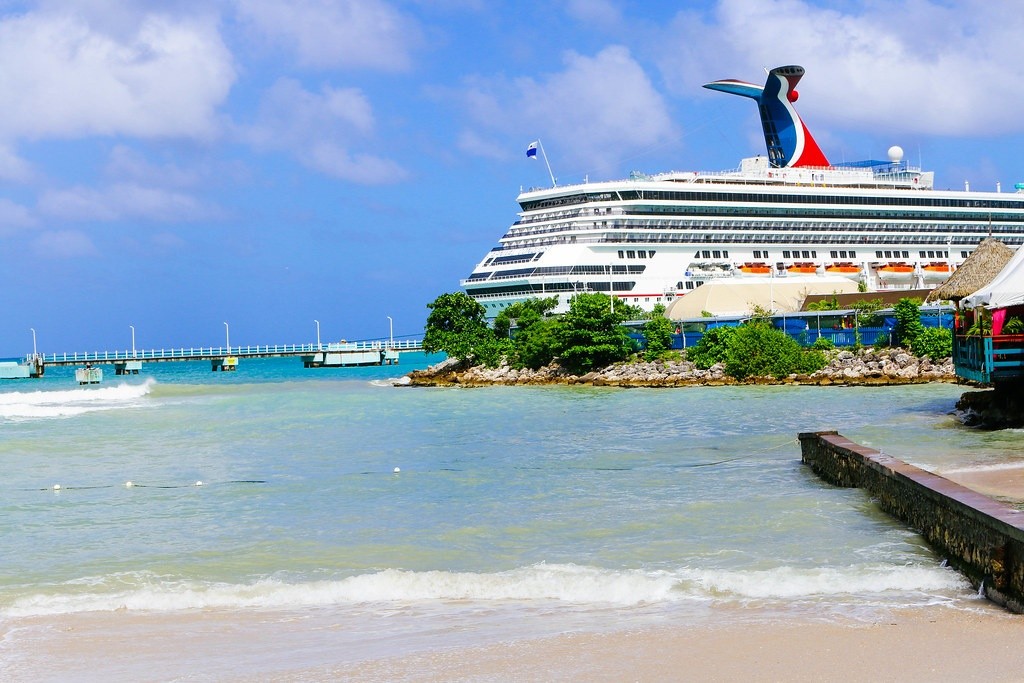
[525,141,537,160]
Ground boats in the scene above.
[459,58,1020,328]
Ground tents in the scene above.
[959,242,1024,311]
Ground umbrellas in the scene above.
[924,236,1017,337]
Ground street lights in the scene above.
[387,316,393,347]
[130,325,136,357]
[223,322,230,354]
[314,319,320,350]
[29,327,38,358]
